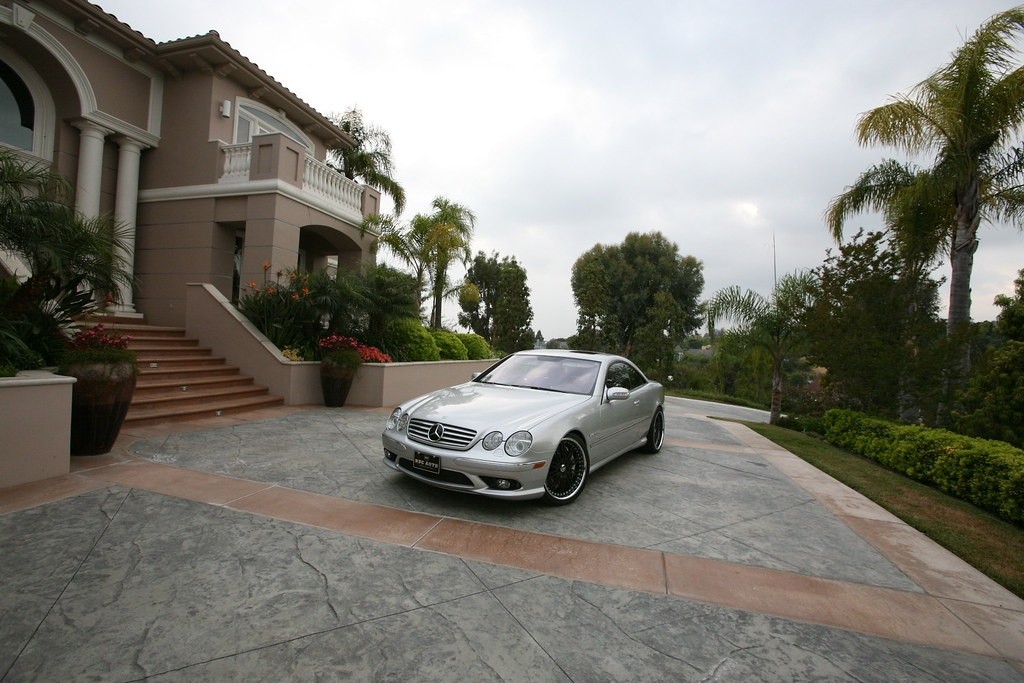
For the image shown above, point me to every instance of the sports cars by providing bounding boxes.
[379,349,666,506]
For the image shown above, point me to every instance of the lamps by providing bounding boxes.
[322,159,326,165]
[219,99,231,119]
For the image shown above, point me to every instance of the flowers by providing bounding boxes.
[69,321,136,364]
[318,333,358,354]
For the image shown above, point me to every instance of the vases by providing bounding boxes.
[57,360,136,457]
[319,348,361,407]
